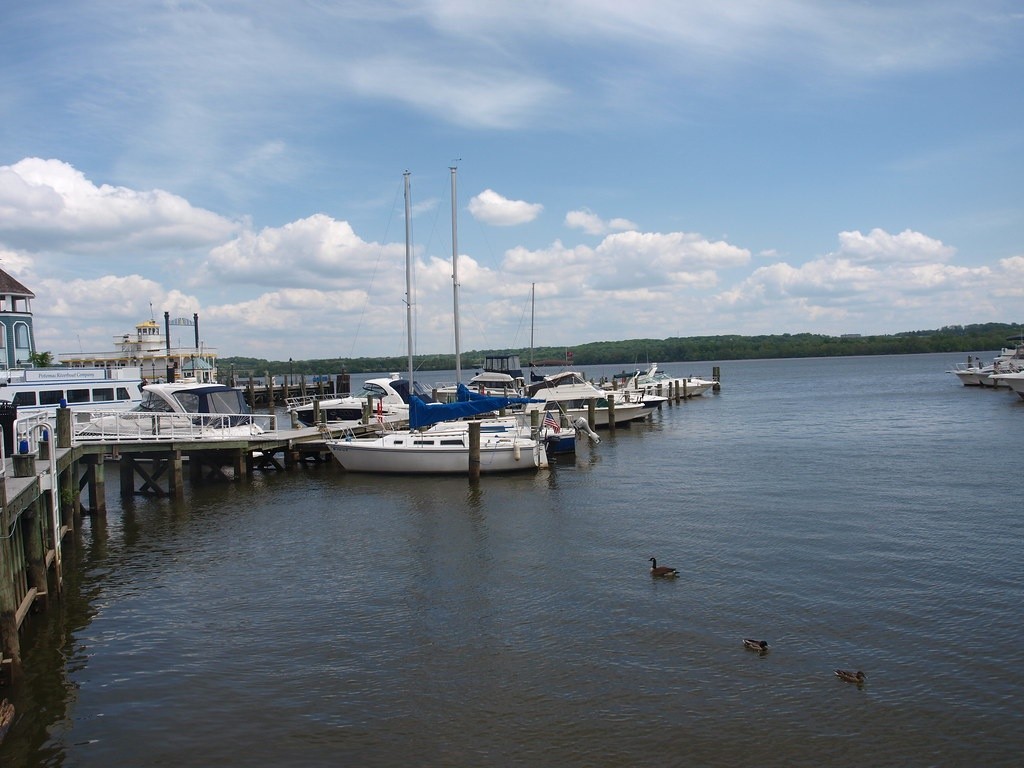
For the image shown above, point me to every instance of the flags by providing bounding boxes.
[544,411,560,433]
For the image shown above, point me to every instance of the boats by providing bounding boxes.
[283,164,719,476]
[946,332,1024,403]
[78,383,267,462]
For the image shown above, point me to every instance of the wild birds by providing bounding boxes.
[742,638,771,652]
[648,556,680,579]
[835,668,867,686]
